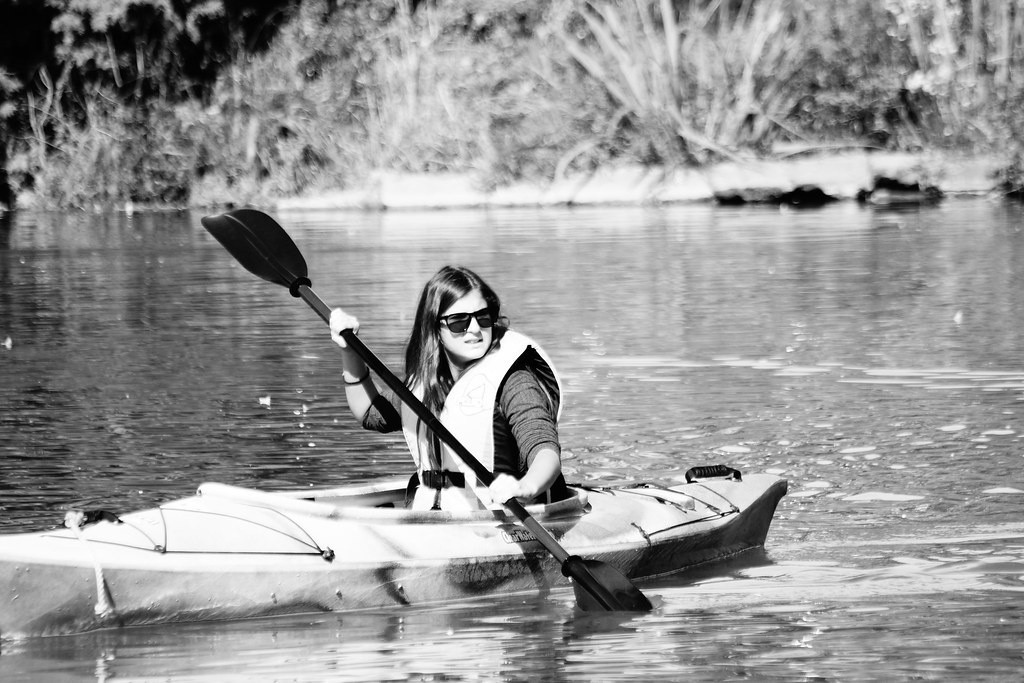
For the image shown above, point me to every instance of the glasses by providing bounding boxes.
[439,307,495,333]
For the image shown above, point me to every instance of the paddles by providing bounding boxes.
[201,207,655,612]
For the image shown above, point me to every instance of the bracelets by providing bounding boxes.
[342,367,370,385]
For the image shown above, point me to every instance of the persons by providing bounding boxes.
[329,265,566,512]
